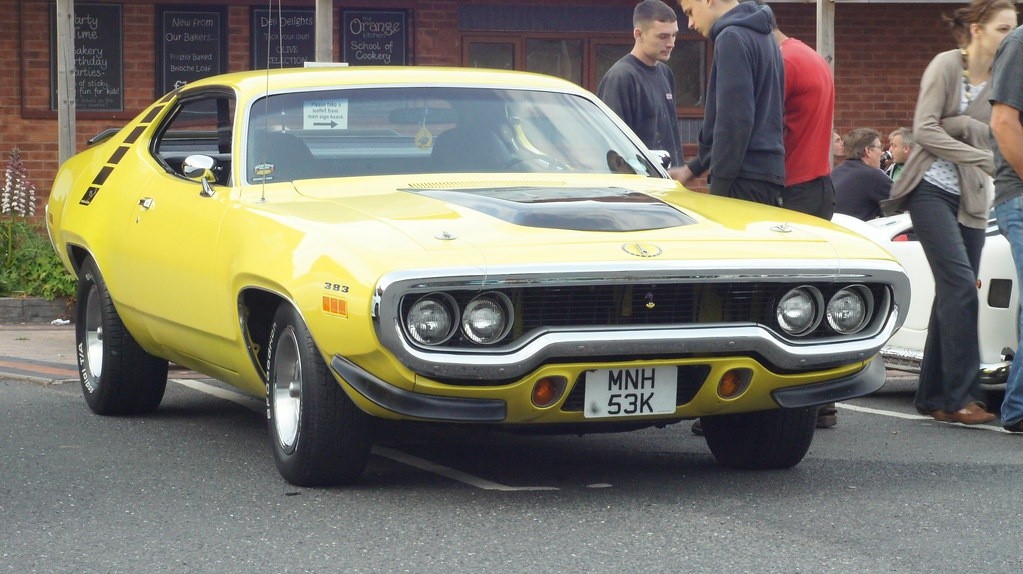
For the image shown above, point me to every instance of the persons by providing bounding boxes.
[596,0,685,180]
[987,25,1023,433]
[830,126,917,222]
[882,1,1018,433]
[667,0,833,221]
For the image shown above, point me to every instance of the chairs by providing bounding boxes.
[427,126,536,174]
[225,132,318,187]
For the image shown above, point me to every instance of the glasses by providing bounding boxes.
[869,145,884,151]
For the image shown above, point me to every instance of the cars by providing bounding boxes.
[45,62,913,487]
[825,210,1021,388]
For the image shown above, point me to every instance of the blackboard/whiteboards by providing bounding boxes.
[342,10,408,65]
[49,2,124,112]
[249,3,316,71]
[154,3,228,101]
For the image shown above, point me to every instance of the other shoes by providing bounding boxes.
[1003,419,1023,432]
[945,401,995,424]
[816,403,837,427]
[692,417,704,435]
[929,409,947,421]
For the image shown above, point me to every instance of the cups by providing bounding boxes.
[880,150,892,165]
